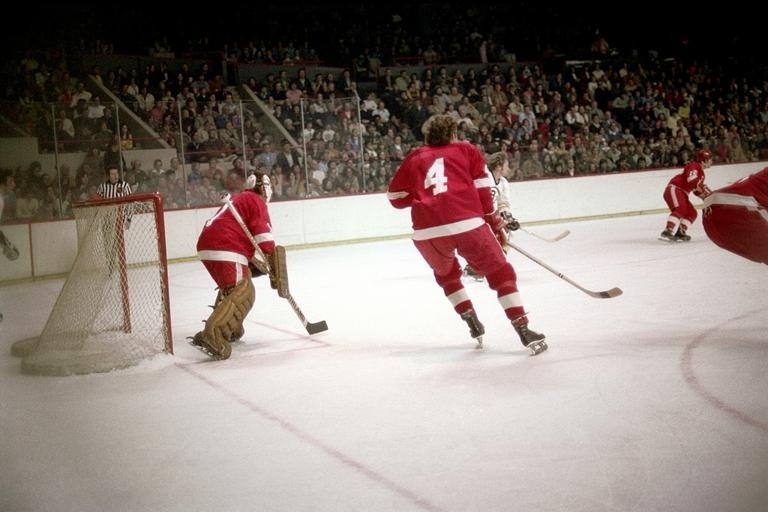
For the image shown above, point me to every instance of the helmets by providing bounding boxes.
[245,172,273,204]
[696,149,713,162]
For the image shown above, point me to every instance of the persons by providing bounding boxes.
[655,149,715,245]
[94,166,134,281]
[385,111,546,350]
[699,165,767,268]
[1,19,767,228]
[185,168,291,360]
[460,149,520,283]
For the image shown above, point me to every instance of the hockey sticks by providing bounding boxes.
[506,242,624,298]
[220,192,328,334]
[518,226,569,245]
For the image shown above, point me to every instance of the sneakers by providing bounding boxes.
[661,230,692,241]
[194,332,231,354]
[471,324,484,338]
[518,327,545,348]
[464,265,479,276]
